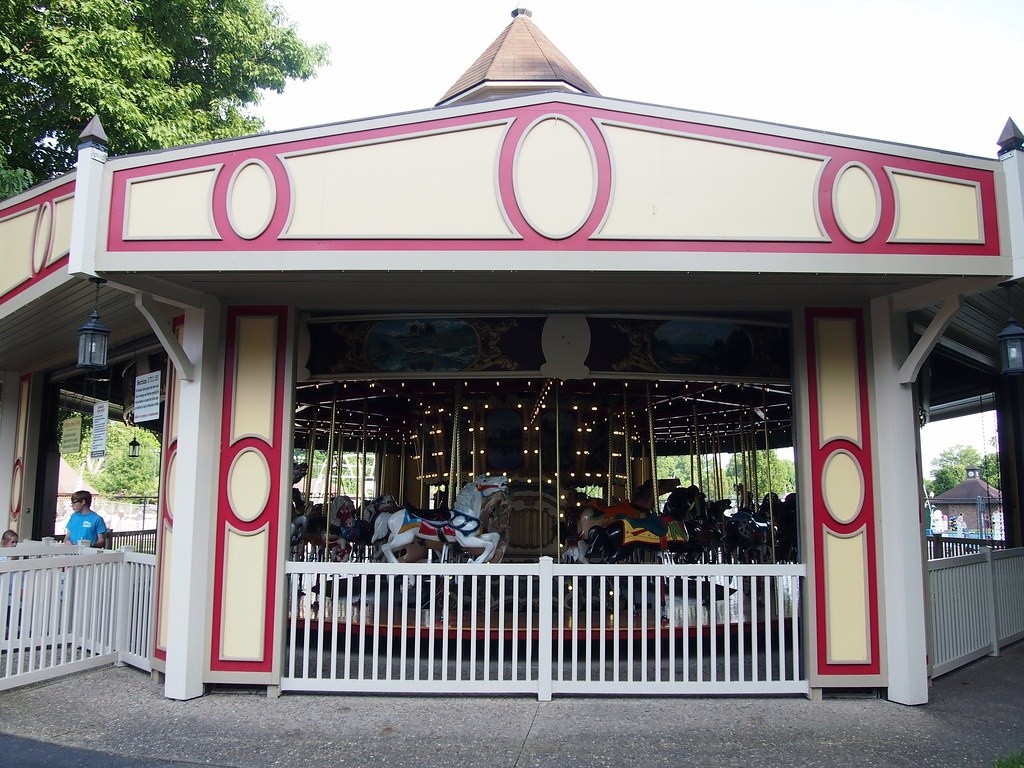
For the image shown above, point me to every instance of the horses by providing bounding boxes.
[553,479,796,624]
[290,462,511,595]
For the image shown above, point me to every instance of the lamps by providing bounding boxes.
[128,438,140,457]
[996,281,1024,376]
[75,278,111,371]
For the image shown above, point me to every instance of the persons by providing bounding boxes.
[63,491,106,567]
[0,531,24,640]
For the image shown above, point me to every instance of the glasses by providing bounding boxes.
[71,500,80,506]
